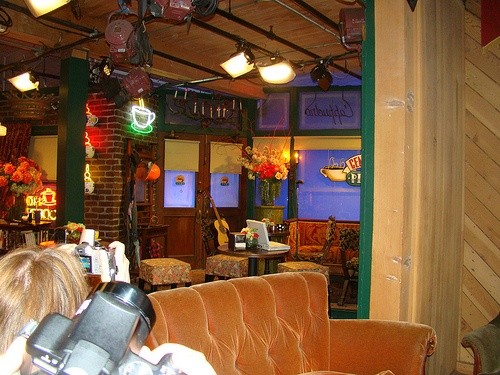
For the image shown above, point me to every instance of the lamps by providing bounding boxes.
[219,42,257,78]
[311,64,334,91]
[258,53,296,85]
[0,0,195,137]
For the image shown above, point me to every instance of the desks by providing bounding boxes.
[218,240,290,276]
[0,219,52,254]
[254,205,285,226]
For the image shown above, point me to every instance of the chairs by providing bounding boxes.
[338,227,359,304]
[461,311,500,375]
[290,215,337,264]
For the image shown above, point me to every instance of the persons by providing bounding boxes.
[0,246,89,375]
[0,315,218,375]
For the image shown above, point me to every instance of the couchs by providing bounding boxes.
[284,218,359,277]
[143,271,438,375]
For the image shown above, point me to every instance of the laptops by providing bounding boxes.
[246,219,290,251]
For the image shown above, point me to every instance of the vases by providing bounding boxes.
[260,181,276,206]
[246,240,258,250]
[14,194,25,220]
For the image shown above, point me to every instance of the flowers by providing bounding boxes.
[238,144,290,186]
[0,156,45,195]
[241,228,258,239]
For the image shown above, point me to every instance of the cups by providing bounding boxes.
[270,225,276,233]
[34,211,41,224]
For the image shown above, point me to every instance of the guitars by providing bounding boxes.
[207,194,231,247]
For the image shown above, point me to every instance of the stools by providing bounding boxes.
[205,254,274,280]
[279,261,331,319]
[138,257,193,294]
